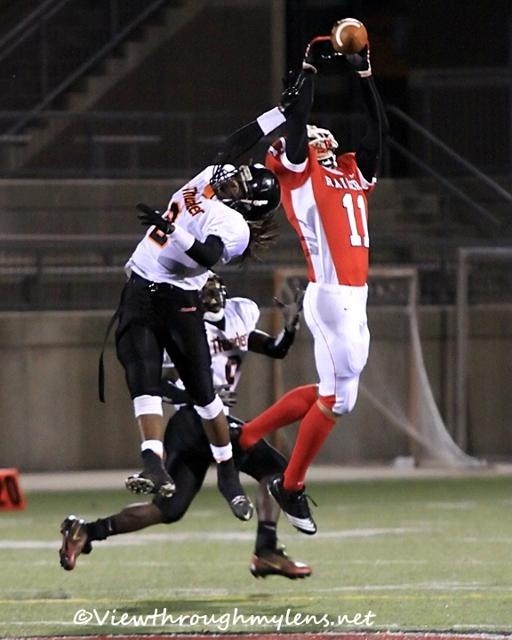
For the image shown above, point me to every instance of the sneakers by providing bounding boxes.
[58,514,92,571]
[266,472,318,535]
[228,422,248,473]
[251,554,311,579]
[216,478,253,521]
[126,469,176,498]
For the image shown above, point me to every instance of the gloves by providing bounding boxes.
[304,35,332,67]
[345,39,372,78]
[278,70,307,114]
[136,201,172,235]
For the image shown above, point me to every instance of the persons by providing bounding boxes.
[57,270,312,580]
[227,37,387,536]
[112,67,306,521]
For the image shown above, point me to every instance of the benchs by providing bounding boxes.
[1,172,511,308]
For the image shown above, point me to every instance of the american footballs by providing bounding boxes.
[332,18,368,54]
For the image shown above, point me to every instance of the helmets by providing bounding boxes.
[196,268,229,311]
[210,158,281,221]
[306,123,339,168]
